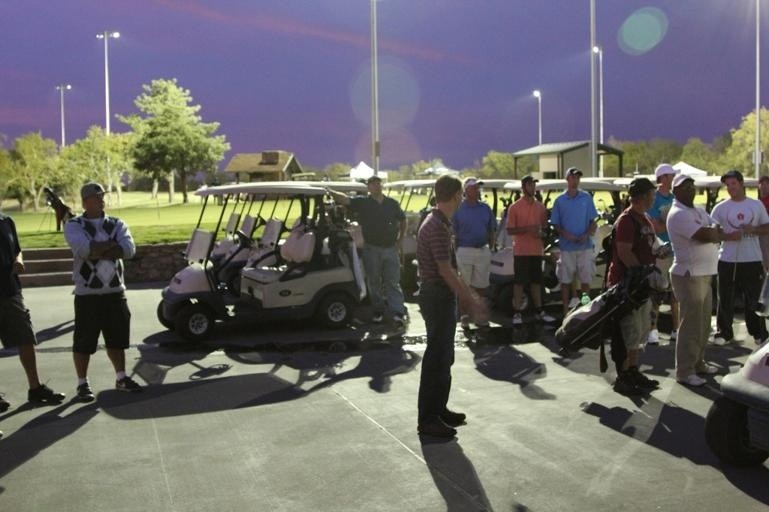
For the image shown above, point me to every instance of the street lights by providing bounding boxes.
[95,30,120,196]
[529,88,545,147]
[591,45,606,181]
[55,82,71,147]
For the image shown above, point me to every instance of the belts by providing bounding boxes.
[371,242,395,248]
[468,242,489,248]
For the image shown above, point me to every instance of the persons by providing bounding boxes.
[646,164,682,344]
[608,178,660,394]
[507,176,556,325]
[452,177,497,330]
[756,176,769,212]
[64,183,142,400]
[710,170,769,344]
[552,167,596,318]
[666,176,726,386]
[416,176,485,436]
[1,213,66,412]
[326,177,407,327]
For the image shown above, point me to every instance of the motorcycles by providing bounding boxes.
[158,182,368,342]
[704,338,769,466]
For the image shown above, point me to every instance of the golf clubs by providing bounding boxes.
[631,264,662,305]
[727,224,743,330]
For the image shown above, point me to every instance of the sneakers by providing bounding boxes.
[439,406,466,422]
[416,417,457,439]
[615,374,647,395]
[677,372,707,386]
[373,312,385,323]
[26,385,66,402]
[634,374,660,388]
[0,395,11,409]
[1,347,18,356]
[535,312,557,322]
[77,382,95,400]
[648,328,660,345]
[714,337,731,346]
[513,312,522,325]
[115,377,142,392]
[462,315,470,329]
[393,312,404,324]
[475,319,490,326]
[671,329,678,340]
[697,360,718,373]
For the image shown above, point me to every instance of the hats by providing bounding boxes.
[670,174,695,191]
[628,178,663,194]
[720,170,743,183]
[521,176,539,184]
[759,176,769,183]
[567,167,584,177]
[81,183,111,199]
[464,177,485,189]
[368,176,382,184]
[656,164,682,177]
